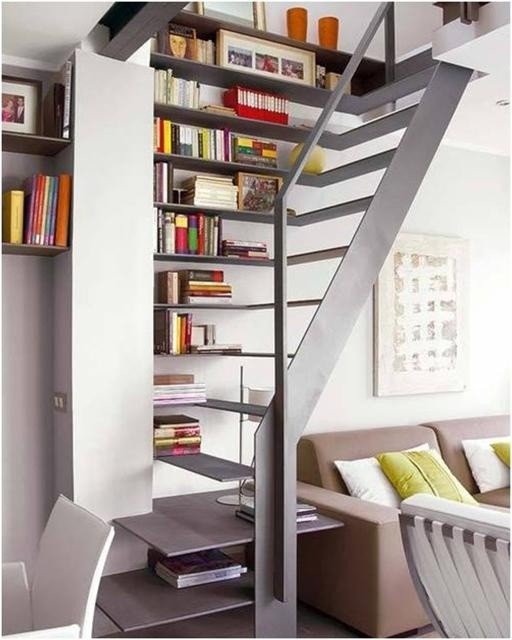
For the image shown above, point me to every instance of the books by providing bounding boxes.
[153,374,207,405]
[154,270,233,305]
[41,58,75,141]
[2,167,73,248]
[152,160,240,209]
[154,551,249,592]
[153,206,268,261]
[234,501,317,529]
[153,415,201,456]
[152,67,291,125]
[154,117,278,168]
[154,310,242,354]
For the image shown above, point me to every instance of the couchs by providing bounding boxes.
[297,414,511,638]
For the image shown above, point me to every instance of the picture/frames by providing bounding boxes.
[2,59,74,139]
[373,229,468,398]
[215,28,317,87]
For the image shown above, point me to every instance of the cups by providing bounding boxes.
[287,7,338,50]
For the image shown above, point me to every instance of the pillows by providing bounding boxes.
[334,436,511,513]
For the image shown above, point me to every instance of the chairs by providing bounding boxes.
[2,493,115,640]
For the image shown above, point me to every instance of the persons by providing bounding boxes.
[3,98,16,122]
[15,95,24,122]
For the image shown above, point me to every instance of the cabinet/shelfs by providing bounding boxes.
[155,6,387,452]
[87,489,347,634]
